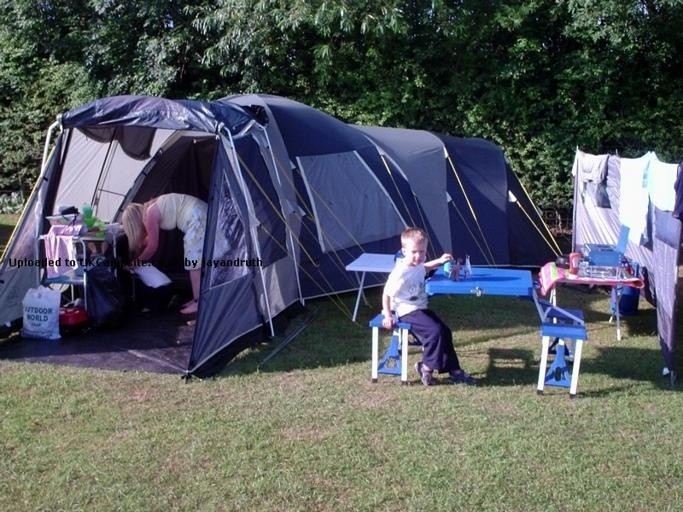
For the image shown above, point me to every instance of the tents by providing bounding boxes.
[0,91,565,384]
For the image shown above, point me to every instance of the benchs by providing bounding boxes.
[370,293,413,386]
[537,300,586,396]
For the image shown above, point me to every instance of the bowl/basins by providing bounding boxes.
[82,201,106,239]
[555,253,569,268]
[582,265,614,277]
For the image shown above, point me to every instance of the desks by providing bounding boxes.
[425,263,545,324]
[538,259,643,343]
[344,252,427,321]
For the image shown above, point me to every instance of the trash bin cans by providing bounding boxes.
[556,255,569,287]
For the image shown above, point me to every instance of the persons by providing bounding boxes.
[383,229,482,386]
[121,193,209,315]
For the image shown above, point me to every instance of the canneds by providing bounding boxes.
[443,259,455,277]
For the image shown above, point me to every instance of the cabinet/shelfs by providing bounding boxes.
[36,223,140,330]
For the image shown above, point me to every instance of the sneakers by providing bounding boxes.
[415,362,432,386]
[448,371,478,384]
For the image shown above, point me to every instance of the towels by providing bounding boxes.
[44,224,87,277]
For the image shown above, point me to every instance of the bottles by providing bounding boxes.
[616,256,638,277]
[441,255,472,279]
